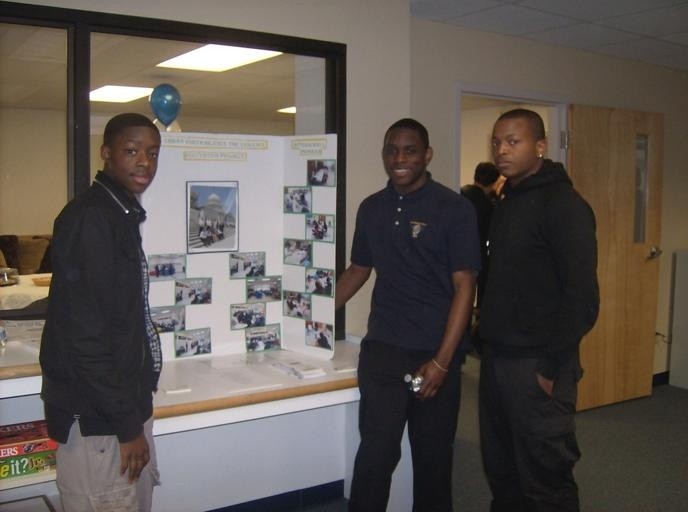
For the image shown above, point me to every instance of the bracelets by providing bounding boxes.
[430,358,452,375]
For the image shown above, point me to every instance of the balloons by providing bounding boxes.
[149,84,181,128]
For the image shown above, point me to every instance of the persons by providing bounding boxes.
[146,164,337,358]
[331,114,479,512]
[461,158,502,359]
[38,108,163,512]
[472,106,600,511]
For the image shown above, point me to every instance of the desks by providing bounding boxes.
[152,338,414,512]
[0,320,48,427]
[0,272,53,317]
